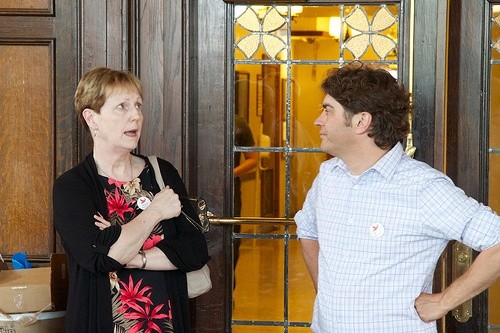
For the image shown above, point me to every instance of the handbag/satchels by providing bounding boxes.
[147,154,212,299]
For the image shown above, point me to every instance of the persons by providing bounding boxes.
[232,118,258,310]
[293,67,500,333]
[52,67,208,333]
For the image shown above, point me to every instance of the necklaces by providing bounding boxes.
[97,158,133,200]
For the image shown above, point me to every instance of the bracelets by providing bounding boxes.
[138,250,147,270]
